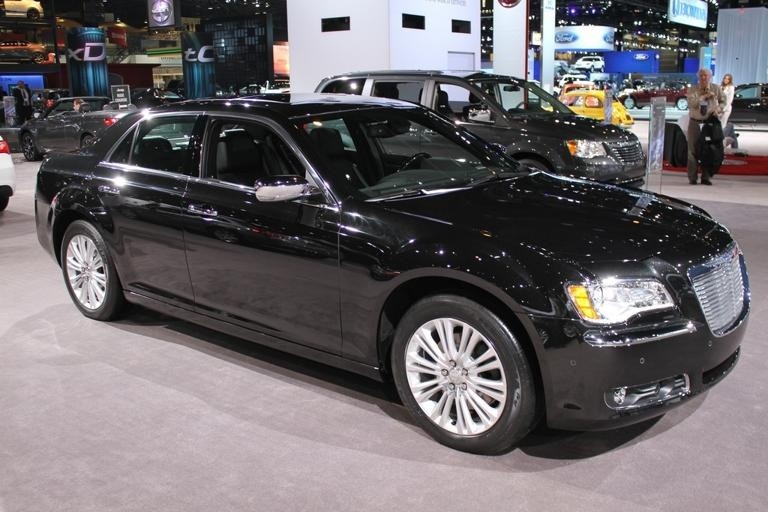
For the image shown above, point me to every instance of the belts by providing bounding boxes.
[690,117,711,123]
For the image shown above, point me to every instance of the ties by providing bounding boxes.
[700,89,707,116]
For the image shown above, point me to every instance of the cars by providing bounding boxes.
[16,96,138,162]
[572,56,607,73]
[0,0,44,21]
[0,38,49,66]
[0,133,16,212]
[31,89,758,461]
[728,81,768,110]
[311,56,693,192]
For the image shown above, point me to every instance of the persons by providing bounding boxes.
[73,99,85,112]
[719,73,734,128]
[686,68,727,186]
[12,81,33,126]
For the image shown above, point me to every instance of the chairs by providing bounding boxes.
[376,85,484,123]
[133,127,380,206]
[79,102,136,111]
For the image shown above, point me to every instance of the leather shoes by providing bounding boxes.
[689,179,712,185]
